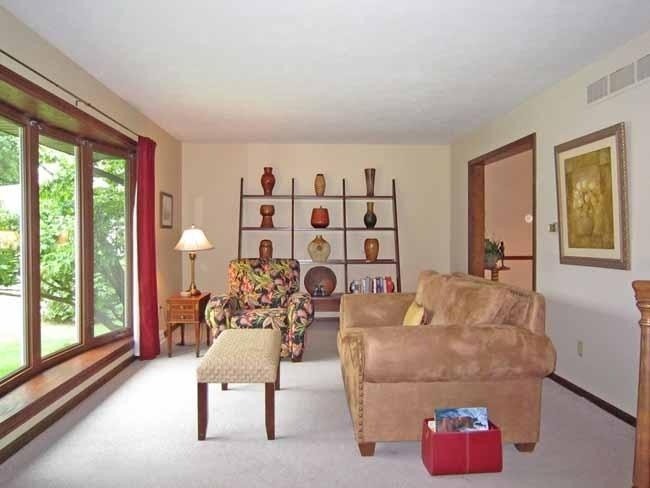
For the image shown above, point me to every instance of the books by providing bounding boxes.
[433,407,489,433]
[353,275,392,293]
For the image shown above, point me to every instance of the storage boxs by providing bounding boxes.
[421,418,503,475]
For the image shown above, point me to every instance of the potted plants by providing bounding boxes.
[486,238,502,268]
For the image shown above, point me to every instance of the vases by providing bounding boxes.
[253,167,379,262]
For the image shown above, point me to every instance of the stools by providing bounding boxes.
[196,328,282,441]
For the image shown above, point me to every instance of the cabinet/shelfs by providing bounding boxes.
[237,178,400,313]
[167,291,211,358]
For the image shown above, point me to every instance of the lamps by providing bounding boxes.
[173,223,214,296]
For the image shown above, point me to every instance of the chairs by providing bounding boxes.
[337,272,556,457]
[205,257,313,362]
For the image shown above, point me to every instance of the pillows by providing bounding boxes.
[414,270,518,326]
[403,302,428,326]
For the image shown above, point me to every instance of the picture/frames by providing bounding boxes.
[159,192,174,227]
[555,122,631,269]
